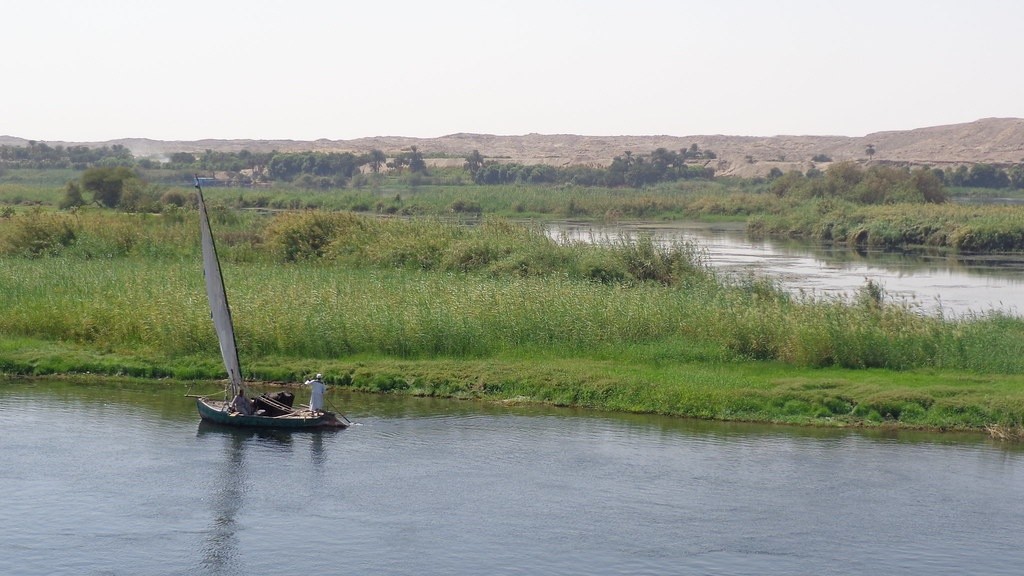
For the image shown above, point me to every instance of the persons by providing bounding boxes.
[232,389,254,415]
[305,374,325,417]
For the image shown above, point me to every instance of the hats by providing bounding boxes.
[316,374,322,378]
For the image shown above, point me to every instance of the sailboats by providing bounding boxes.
[188,173,347,428]
